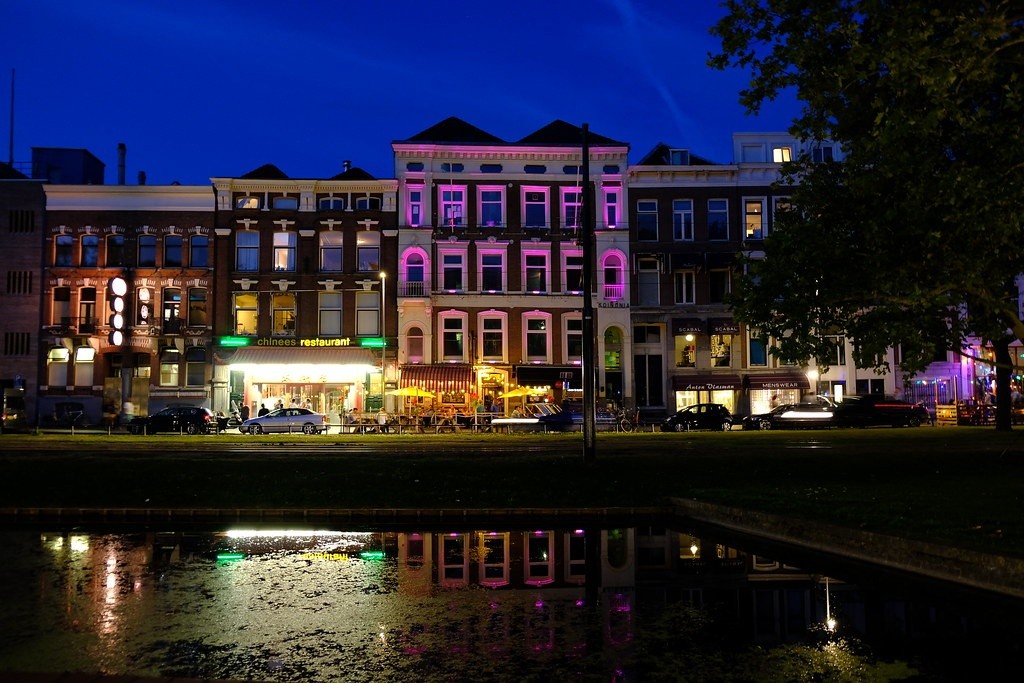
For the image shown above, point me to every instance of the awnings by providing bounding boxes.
[671,373,741,392]
[400,363,473,393]
[743,372,811,390]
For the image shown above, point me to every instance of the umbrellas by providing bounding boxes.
[498,386,548,416]
[385,386,436,418]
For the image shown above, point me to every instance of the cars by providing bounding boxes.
[660,403,733,431]
[741,403,833,429]
[238,407,327,434]
[539,403,617,432]
[127,406,211,435]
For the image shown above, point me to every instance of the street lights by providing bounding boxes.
[379,271,389,411]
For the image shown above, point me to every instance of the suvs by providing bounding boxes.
[837,395,928,427]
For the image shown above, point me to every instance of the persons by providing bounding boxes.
[415,398,499,433]
[510,405,543,434]
[768,395,778,411]
[348,407,389,434]
[802,391,817,403]
[206,397,313,432]
[916,388,1024,426]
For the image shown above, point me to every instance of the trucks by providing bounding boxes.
[16,395,103,428]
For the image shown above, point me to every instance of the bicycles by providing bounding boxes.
[618,411,638,433]
[916,395,934,427]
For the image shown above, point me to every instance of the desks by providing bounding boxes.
[353,417,379,433]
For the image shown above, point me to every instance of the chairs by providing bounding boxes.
[342,415,465,434]
